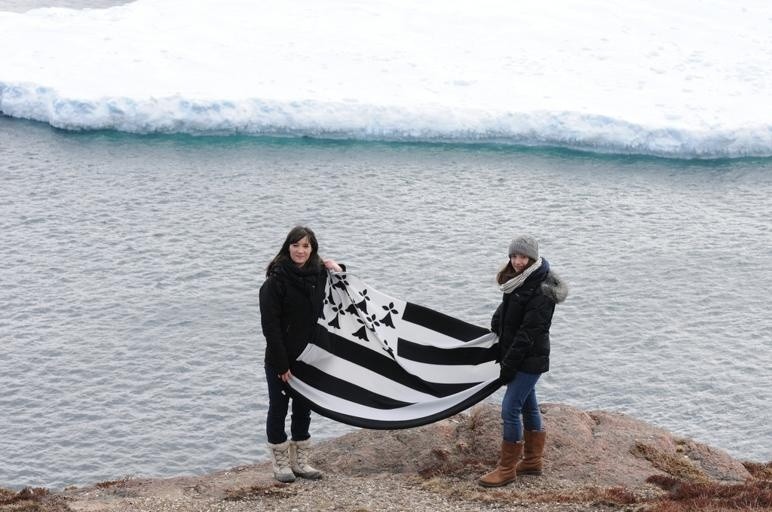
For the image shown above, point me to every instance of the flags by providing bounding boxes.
[286,260,506,433]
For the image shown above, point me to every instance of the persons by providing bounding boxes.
[259,223,349,481]
[473,235,569,486]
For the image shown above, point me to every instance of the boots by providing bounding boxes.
[267,439,296,482]
[478,439,526,487]
[515,429,547,476]
[289,436,325,479]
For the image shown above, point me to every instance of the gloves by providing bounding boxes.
[491,317,499,333]
[499,365,515,385]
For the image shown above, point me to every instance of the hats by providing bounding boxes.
[508,234,538,262]
